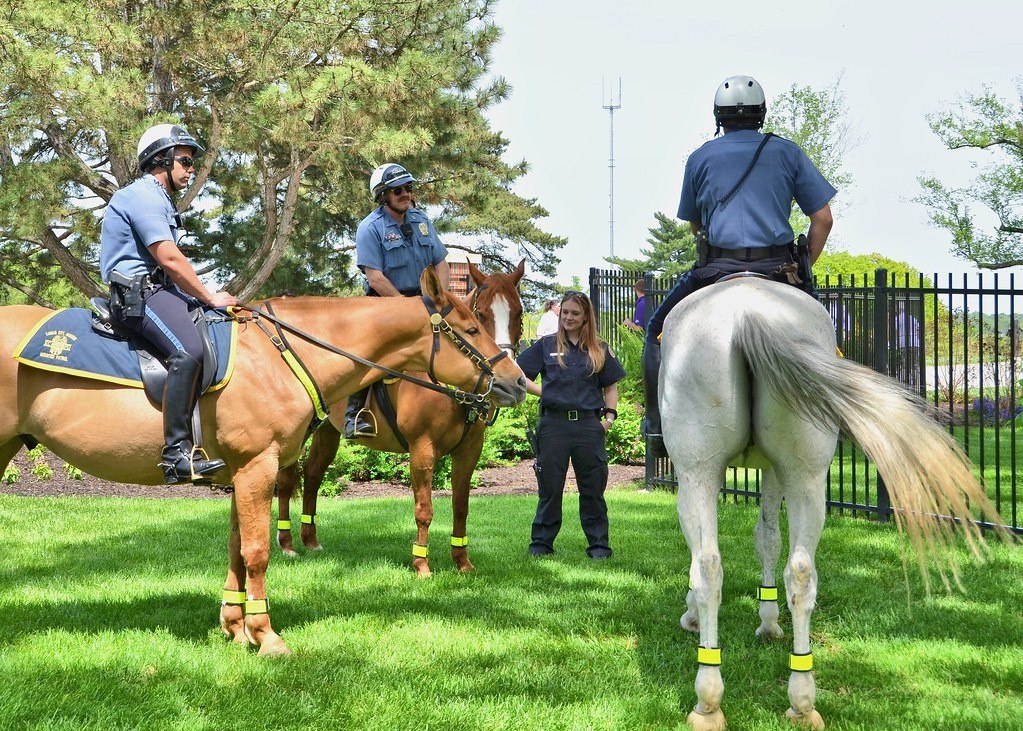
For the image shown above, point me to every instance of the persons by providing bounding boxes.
[820,291,855,342]
[624,279,659,333]
[100,124,237,485]
[516,291,627,559]
[536,299,561,340]
[888,300,920,389]
[640,76,838,458]
[342,163,450,439]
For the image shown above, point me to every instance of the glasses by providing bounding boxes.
[173,156,193,167]
[564,291,586,297]
[391,185,413,196]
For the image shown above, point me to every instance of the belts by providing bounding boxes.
[543,407,597,421]
[708,244,790,261]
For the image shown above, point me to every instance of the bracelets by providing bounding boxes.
[606,418,613,426]
[605,408,618,420]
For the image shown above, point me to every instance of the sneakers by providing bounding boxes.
[641,419,668,457]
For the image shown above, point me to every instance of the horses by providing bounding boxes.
[0,256,526,657]
[657,273,990,731]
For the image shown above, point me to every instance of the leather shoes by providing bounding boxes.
[160,443,225,485]
[344,415,373,439]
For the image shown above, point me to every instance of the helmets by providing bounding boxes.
[713,75,767,136]
[138,124,204,169]
[369,163,417,202]
